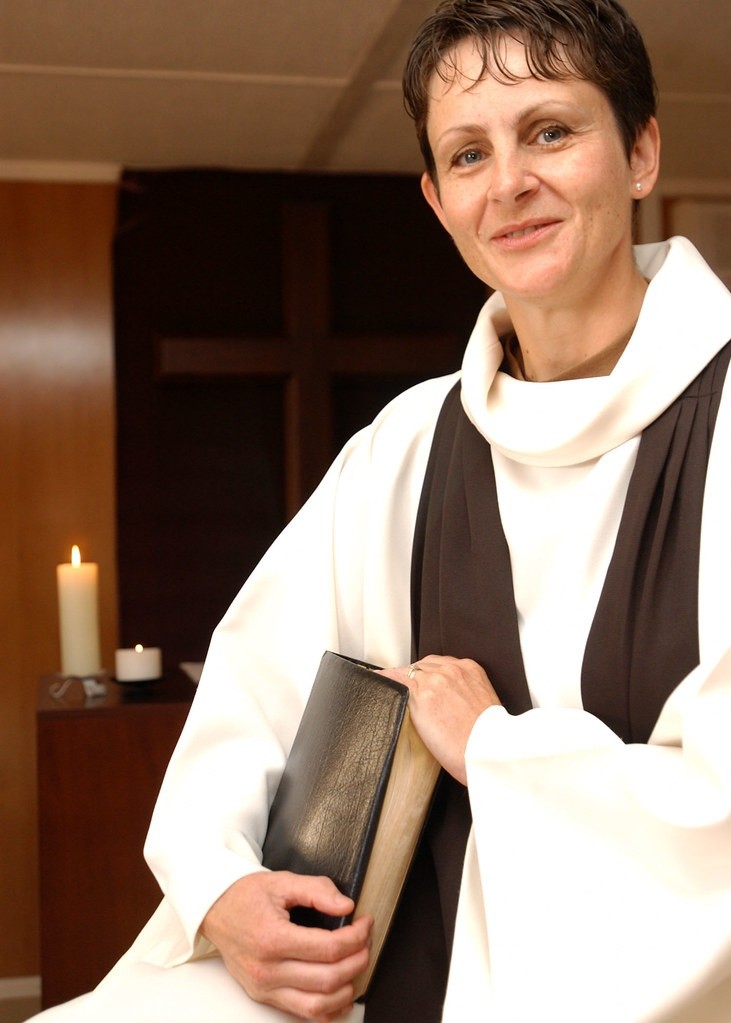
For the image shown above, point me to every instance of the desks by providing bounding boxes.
[36,673,192,1015]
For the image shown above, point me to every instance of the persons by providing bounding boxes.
[26,0,731,1023]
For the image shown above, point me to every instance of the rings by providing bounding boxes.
[408,667,420,677]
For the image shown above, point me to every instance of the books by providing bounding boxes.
[261,649,447,1005]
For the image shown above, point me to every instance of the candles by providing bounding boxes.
[115,642,163,680]
[56,545,100,677]
